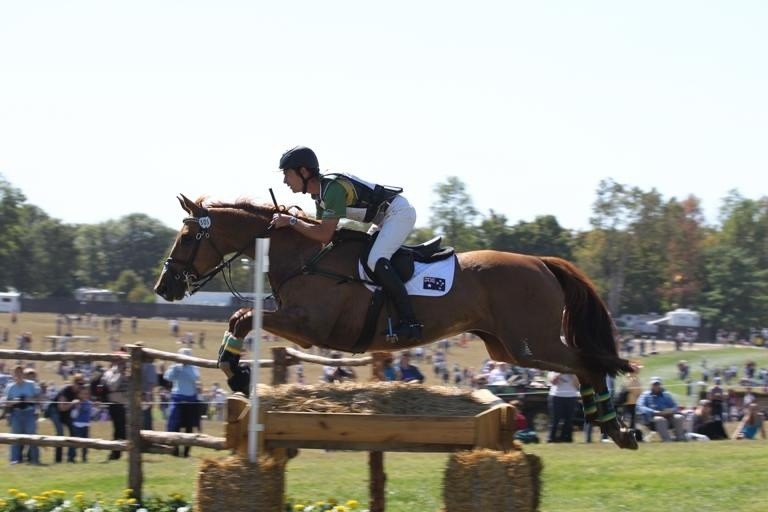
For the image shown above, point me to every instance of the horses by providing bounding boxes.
[153,192,641,451]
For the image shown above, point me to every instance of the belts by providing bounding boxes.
[378,196,396,212]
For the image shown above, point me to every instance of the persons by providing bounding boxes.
[267,338,357,384]
[0,309,252,464]
[268,144,424,347]
[621,327,768,441]
[383,333,615,442]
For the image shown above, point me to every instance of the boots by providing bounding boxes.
[373,257,422,342]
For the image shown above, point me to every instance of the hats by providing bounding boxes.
[73,373,84,385]
[651,377,661,385]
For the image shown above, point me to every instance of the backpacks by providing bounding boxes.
[91,369,107,403]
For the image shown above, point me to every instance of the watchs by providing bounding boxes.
[289,216,297,229]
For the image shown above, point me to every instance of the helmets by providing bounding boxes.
[279,145,319,169]
